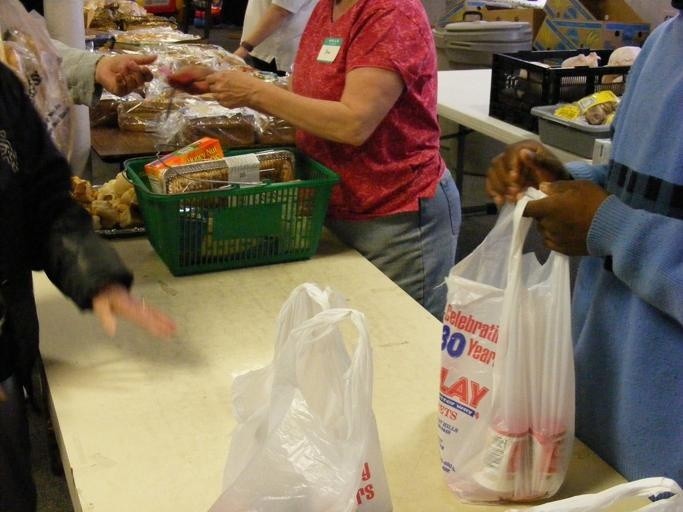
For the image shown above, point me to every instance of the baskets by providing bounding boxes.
[124,141,344,277]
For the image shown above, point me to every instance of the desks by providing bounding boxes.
[436,68,592,241]
[34,234,660,512]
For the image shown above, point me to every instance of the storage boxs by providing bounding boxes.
[531,102,612,160]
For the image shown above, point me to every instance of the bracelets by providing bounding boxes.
[241,40,254,53]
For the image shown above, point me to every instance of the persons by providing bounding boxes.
[49,0,157,109]
[233,0,316,78]
[165,0,462,323]
[487,0,682,512]
[1,60,175,512]
